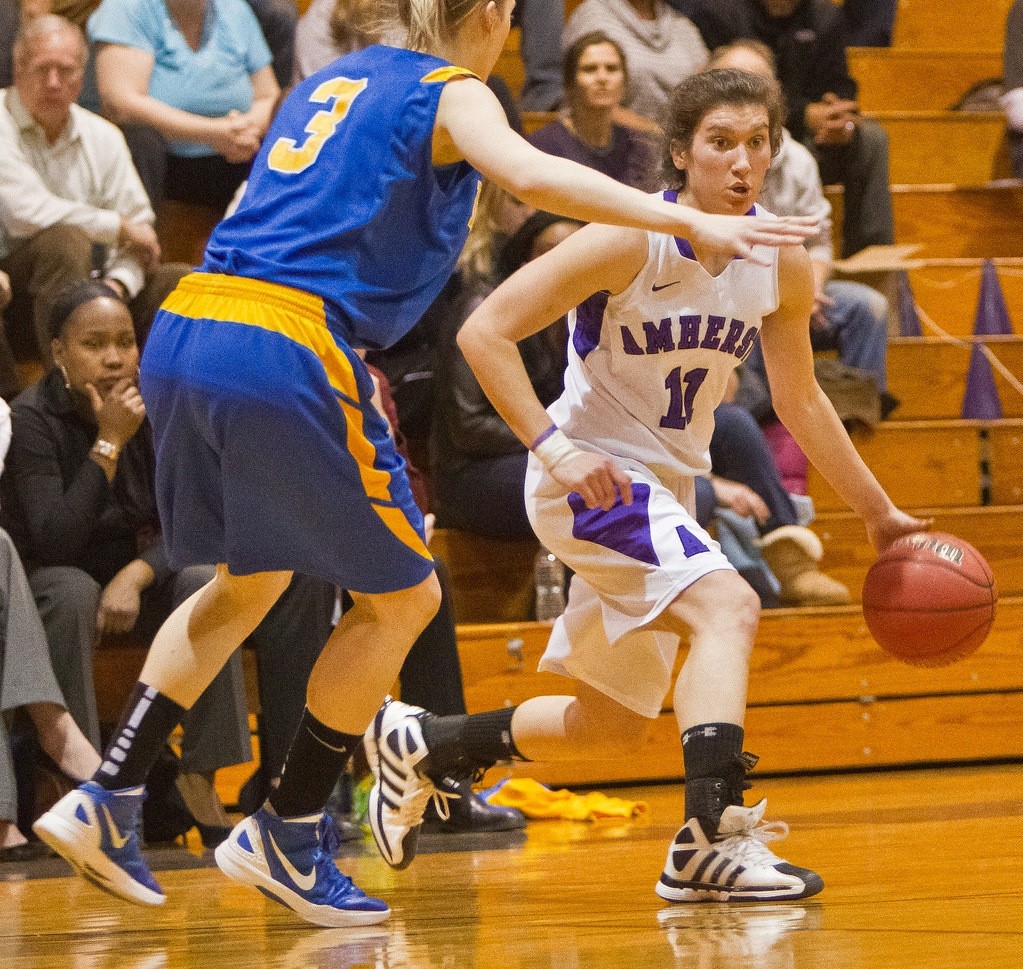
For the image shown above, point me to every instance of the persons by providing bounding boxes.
[7,283,253,852]
[0,0,893,611]
[33,0,821,930]
[364,67,938,904]
[0,400,107,857]
[256,342,527,835]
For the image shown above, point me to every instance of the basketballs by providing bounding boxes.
[861,529,1000,669]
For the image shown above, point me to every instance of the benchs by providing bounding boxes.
[0,0,1023,814]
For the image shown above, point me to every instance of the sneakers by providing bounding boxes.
[657,905,821,960]
[283,926,393,969]
[32,780,168,908]
[653,798,825,902]
[361,694,462,872]
[215,805,390,928]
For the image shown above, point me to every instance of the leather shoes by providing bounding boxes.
[422,784,526,832]
[324,806,366,841]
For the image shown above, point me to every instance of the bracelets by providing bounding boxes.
[94,438,120,462]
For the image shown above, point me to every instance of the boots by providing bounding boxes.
[756,523,851,608]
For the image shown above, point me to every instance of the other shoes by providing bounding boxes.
[0,839,33,863]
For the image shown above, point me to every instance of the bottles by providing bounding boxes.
[534,542,566,624]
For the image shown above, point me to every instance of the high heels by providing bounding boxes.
[169,779,233,850]
